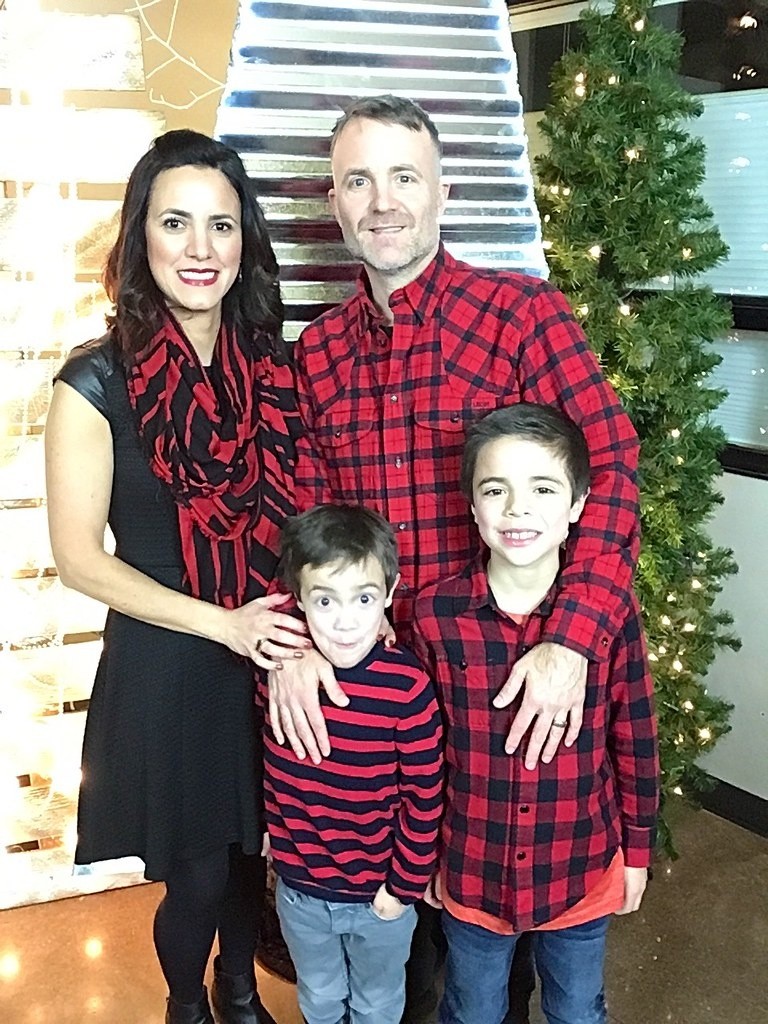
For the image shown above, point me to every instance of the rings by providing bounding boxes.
[256,638,268,650]
[552,721,567,728]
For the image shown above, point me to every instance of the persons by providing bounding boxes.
[408,402,661,1024]
[255,502,446,1024]
[44,128,296,1024]
[296,94,644,1024]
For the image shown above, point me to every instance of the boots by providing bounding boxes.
[211,957,276,1024]
[164,986,214,1024]
